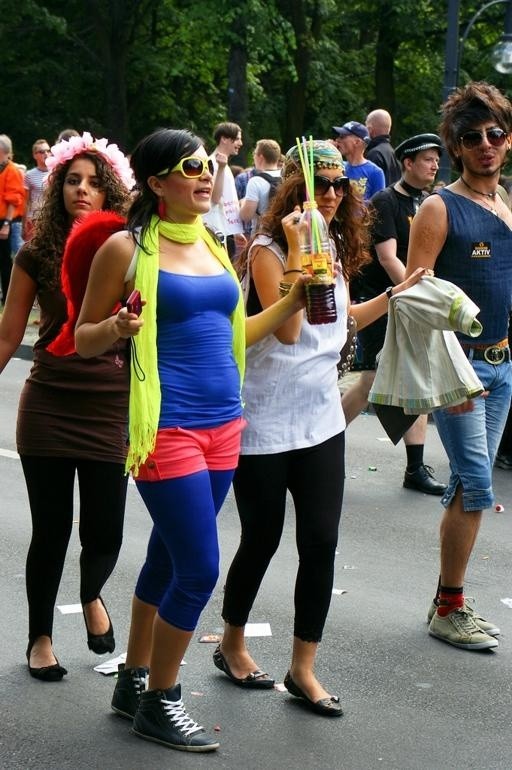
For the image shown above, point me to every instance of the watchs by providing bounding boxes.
[384,286,394,302]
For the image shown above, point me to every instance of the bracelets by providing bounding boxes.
[281,269,305,277]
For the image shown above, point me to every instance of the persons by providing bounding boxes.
[341,129,448,499]
[212,137,433,720]
[2,130,136,683]
[404,80,512,653]
[73,125,314,755]
[0,78,404,311]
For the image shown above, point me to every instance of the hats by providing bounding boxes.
[394,134,441,161]
[333,121,371,144]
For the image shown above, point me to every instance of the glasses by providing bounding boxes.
[312,175,350,198]
[158,157,214,178]
[461,129,506,149]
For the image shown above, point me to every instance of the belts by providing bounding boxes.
[461,348,511,364]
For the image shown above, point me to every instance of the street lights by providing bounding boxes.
[435,0,512,187]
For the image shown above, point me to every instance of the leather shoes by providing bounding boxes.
[214,643,274,688]
[284,670,342,716]
[403,464,448,496]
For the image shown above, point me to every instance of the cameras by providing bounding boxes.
[126,291,142,318]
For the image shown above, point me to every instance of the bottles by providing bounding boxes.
[299,201,336,324]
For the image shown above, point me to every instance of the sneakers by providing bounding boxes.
[26,646,67,681]
[429,607,498,649]
[429,599,500,636]
[131,684,220,752]
[83,595,115,654]
[111,663,150,719]
